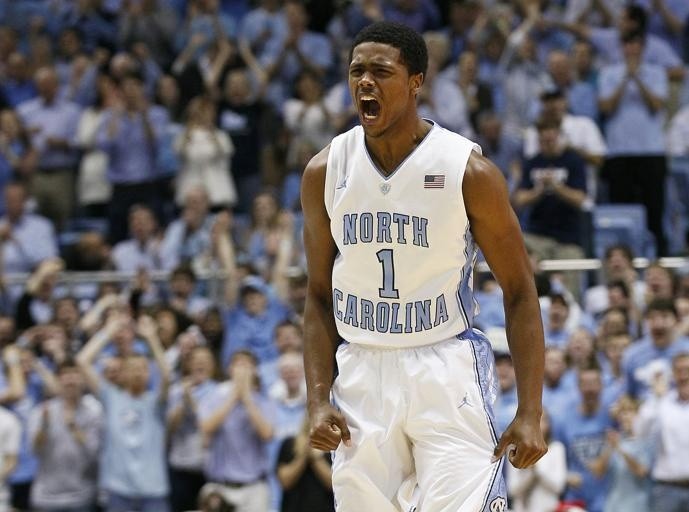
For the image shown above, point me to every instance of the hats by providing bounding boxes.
[482,326,509,355]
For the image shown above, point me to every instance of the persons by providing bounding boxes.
[1,2,360,507]
[298,21,550,509]
[385,4,687,511]
